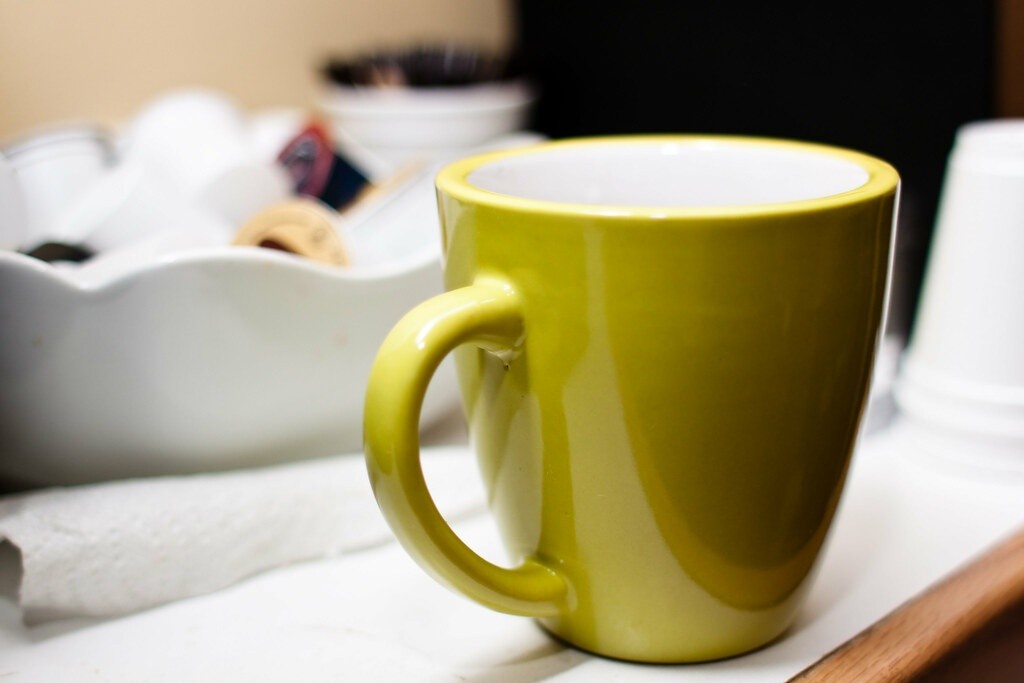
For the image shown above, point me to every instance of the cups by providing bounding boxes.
[364,135,901,668]
[313,93,528,178]
[895,121,1024,445]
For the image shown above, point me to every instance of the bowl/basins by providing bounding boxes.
[0,133,551,486]
